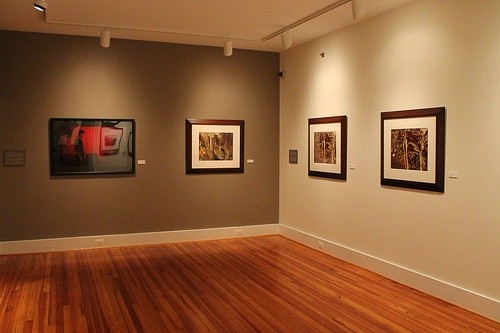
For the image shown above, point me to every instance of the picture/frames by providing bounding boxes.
[185,119,244,174]
[308,116,346,181]
[381,107,445,193]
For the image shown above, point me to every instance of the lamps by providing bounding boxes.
[224,38,232,56]
[281,30,292,50]
[352,0,365,21]
[33,0,48,12]
[100,27,110,48]
